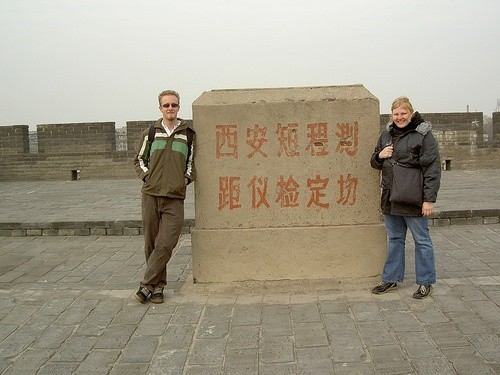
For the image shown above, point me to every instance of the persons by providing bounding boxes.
[370,96,440,299]
[134,89,195,304]
